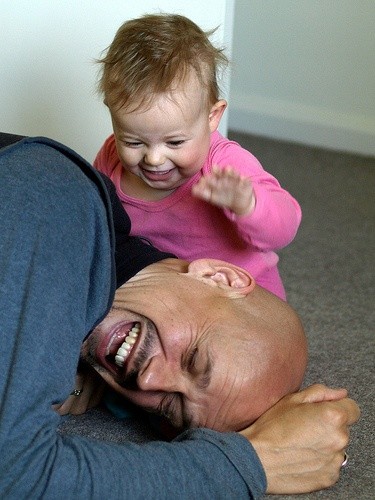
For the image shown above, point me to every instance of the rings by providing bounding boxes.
[340,453,349,468]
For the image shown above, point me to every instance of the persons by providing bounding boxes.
[1,132,361,500]
[92,14,301,301]
